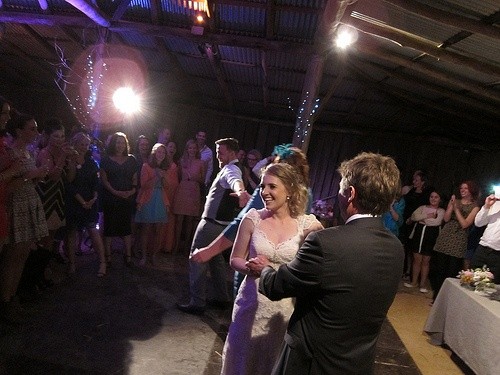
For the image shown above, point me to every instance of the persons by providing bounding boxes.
[245,152,405,375]
[1,95,500,325]
[221,162,326,375]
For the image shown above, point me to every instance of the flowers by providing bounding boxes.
[456,265,494,295]
[311,199,334,220]
[270,142,295,160]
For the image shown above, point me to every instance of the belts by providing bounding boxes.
[206,218,227,227]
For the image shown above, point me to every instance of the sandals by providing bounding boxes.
[96,262,108,277]
[68,261,78,274]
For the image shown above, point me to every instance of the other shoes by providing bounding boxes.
[176,303,205,315]
[126,255,132,266]
[404,282,419,287]
[107,254,113,265]
[207,300,232,310]
[420,286,428,292]
[136,245,156,264]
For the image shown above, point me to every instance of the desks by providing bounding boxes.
[423,278,500,375]
